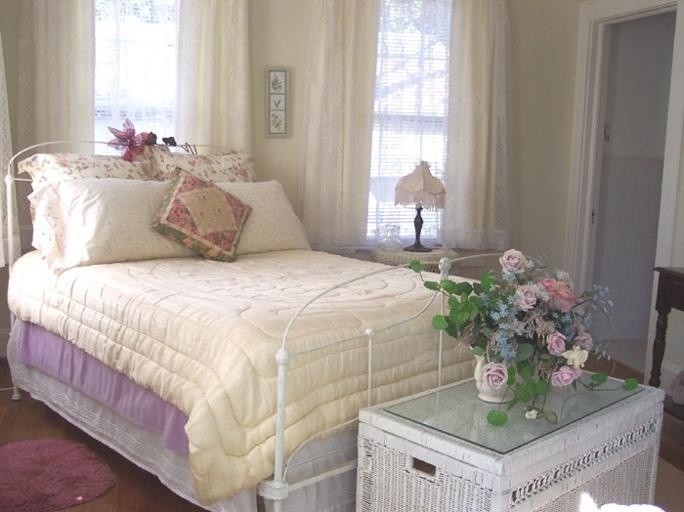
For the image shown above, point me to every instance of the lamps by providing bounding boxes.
[393,158,449,250]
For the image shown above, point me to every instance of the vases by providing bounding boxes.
[466,343,523,402]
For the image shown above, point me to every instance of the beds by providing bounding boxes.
[5,144,518,512]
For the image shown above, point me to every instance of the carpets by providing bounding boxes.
[1,438,116,511]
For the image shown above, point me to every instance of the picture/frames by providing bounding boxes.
[263,65,290,137]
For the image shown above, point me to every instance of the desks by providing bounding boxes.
[372,247,459,276]
[355,362,666,512]
[649,265,684,390]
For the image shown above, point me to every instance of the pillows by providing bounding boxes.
[152,168,253,263]
[219,179,313,254]
[15,152,154,181]
[154,144,257,183]
[28,177,200,276]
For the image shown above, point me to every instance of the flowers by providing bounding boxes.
[406,248,639,425]
[108,118,197,161]
